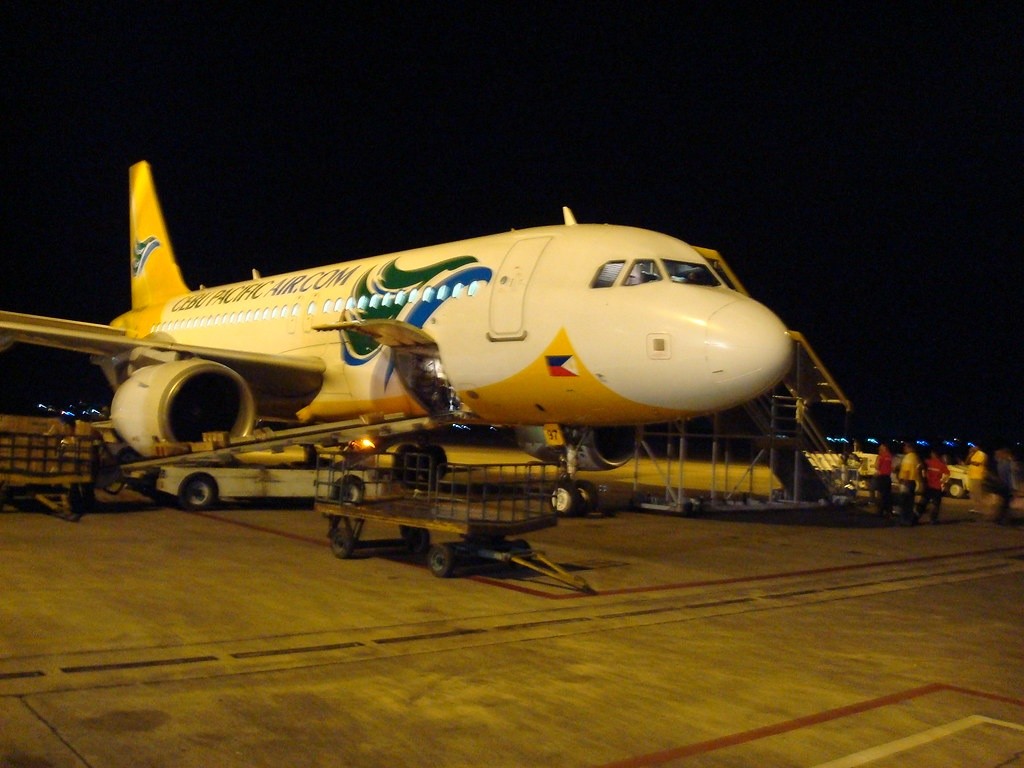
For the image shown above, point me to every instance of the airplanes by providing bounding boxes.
[2,160,795,596]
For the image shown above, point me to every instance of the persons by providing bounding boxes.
[869,443,893,518]
[994,449,1019,526]
[55,417,81,519]
[965,441,988,514]
[896,441,950,527]
